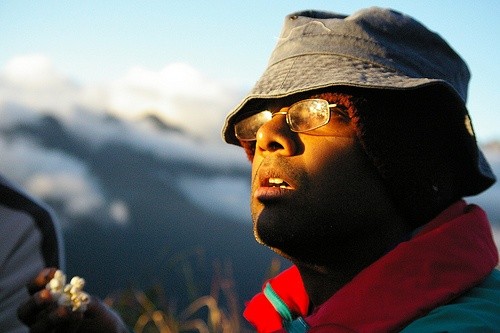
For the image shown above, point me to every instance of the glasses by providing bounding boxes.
[232,98,350,142]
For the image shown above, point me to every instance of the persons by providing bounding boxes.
[17,7,500,333]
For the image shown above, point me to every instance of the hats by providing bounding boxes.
[221,6,498,198]
